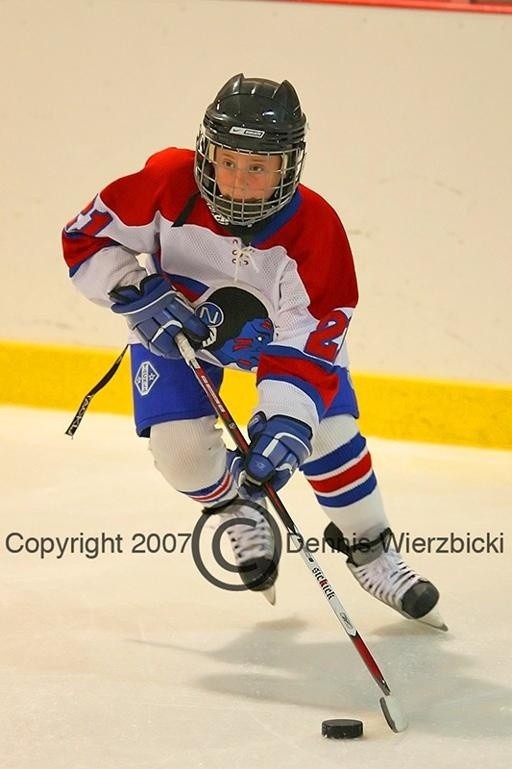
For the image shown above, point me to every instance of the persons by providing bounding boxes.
[60,72,441,621]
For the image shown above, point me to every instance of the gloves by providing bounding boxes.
[109,273,210,361]
[225,412,317,501]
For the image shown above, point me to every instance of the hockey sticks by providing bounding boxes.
[138,252,407,730]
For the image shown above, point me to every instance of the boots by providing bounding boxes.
[323,519,440,621]
[196,487,279,591]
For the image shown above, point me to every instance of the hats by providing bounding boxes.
[199,73,307,180]
